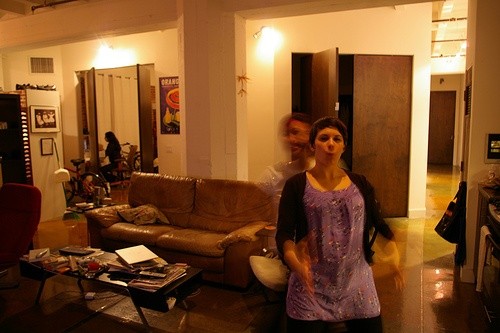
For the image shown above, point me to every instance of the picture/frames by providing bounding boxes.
[29,105,61,133]
[40,138,53,155]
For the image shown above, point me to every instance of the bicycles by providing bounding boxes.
[63,159,109,204]
[119,143,141,170]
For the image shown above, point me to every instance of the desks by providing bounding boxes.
[472,183,500,324]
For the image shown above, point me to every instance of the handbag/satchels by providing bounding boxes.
[434,189,461,243]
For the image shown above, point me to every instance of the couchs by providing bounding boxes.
[85,171,273,293]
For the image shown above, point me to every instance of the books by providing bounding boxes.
[104,244,190,293]
[59,244,95,256]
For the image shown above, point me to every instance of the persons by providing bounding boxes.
[35,112,55,127]
[249,113,404,333]
[99,132,121,182]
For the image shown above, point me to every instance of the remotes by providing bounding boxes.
[139,271,166,279]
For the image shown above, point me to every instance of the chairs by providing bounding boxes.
[0,183,41,309]
[110,145,137,189]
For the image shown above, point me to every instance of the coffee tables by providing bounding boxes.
[19,247,204,333]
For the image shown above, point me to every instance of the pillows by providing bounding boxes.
[117,203,171,225]
